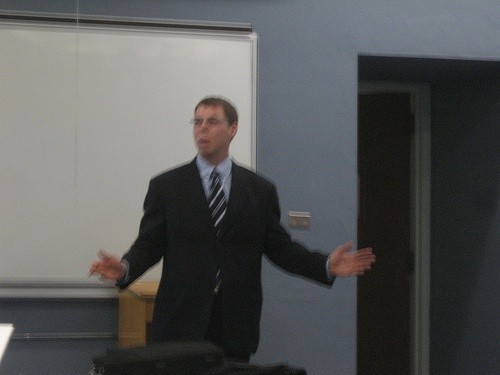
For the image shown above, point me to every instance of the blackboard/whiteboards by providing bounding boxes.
[0,8,259,304]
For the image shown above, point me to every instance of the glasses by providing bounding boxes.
[190,115,226,129]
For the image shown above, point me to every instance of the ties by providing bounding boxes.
[206,166,230,239]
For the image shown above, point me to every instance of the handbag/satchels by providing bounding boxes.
[90,340,229,375]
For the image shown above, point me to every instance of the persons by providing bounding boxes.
[88,96,376,364]
